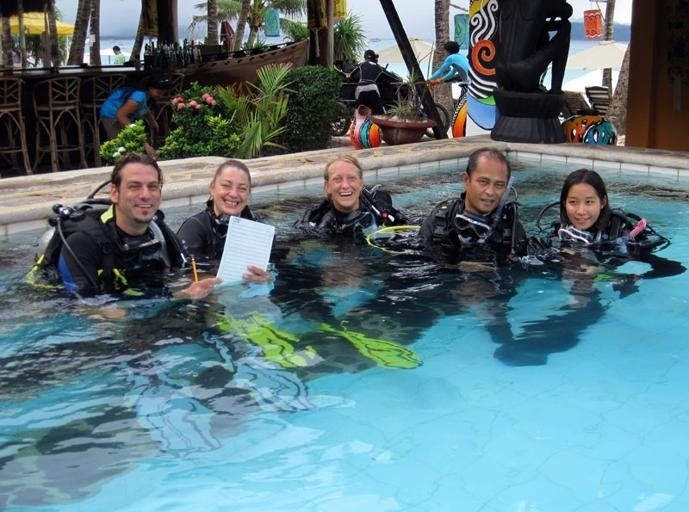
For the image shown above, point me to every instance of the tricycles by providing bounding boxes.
[326,58,450,138]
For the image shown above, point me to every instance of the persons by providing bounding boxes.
[493,1,573,97]
[529,169,652,248]
[113,45,126,66]
[418,147,532,259]
[100,68,172,162]
[428,40,470,138]
[176,160,291,262]
[49,152,271,299]
[350,49,404,116]
[293,153,411,237]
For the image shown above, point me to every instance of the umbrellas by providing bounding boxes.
[357,37,437,64]
[0,11,75,37]
[564,38,631,69]
[220,19,236,54]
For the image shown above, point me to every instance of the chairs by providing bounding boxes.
[1,72,184,175]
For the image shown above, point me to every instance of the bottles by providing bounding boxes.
[143,37,202,70]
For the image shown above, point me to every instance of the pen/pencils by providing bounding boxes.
[191,255,198,282]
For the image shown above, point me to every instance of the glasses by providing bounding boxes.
[558,222,591,247]
[453,214,491,238]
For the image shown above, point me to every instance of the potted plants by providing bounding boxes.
[372,66,439,145]
